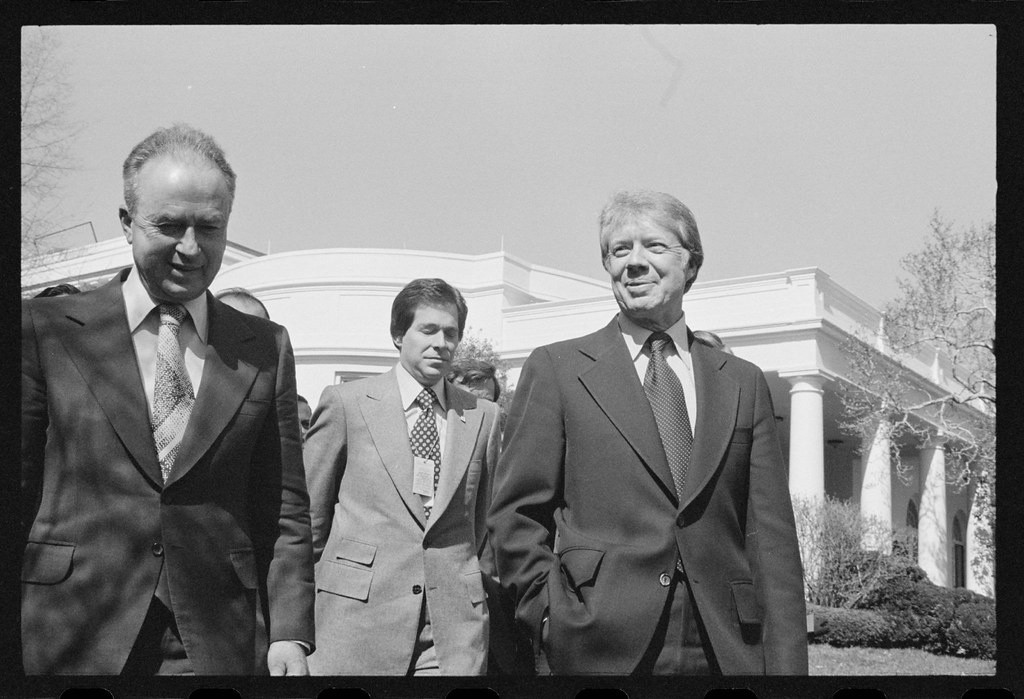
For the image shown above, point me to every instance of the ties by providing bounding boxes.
[409,388,442,521]
[643,332,693,573]
[154,303,195,614]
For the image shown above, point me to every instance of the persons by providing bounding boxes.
[32,277,500,440]
[15,123,317,675]
[298,277,499,677]
[489,190,811,676]
